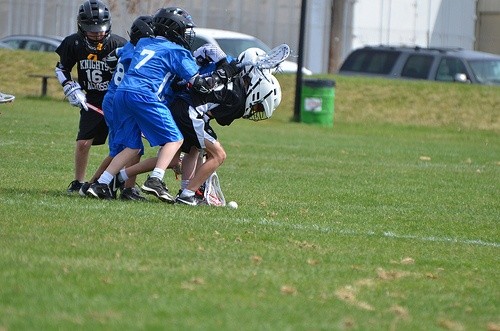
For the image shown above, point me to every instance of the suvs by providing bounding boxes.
[338,43,500,86]
[184,27,313,76]
[0,34,65,52]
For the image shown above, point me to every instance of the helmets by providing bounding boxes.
[235,47,283,119]
[130,15,154,47]
[152,7,197,50]
[77,0,112,32]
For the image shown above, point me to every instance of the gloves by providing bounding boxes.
[62,81,89,112]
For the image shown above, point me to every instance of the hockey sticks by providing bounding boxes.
[177,43,290,85]
[84,101,146,139]
[203,170,226,207]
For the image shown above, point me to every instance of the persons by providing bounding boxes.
[54,0,129,193]
[79,8,282,206]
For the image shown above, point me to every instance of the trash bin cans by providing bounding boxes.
[300,79,336,127]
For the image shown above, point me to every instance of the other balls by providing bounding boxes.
[227,201,238,210]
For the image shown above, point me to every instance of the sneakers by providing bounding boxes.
[67,175,207,206]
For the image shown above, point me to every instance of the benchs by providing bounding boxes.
[28,73,78,96]
